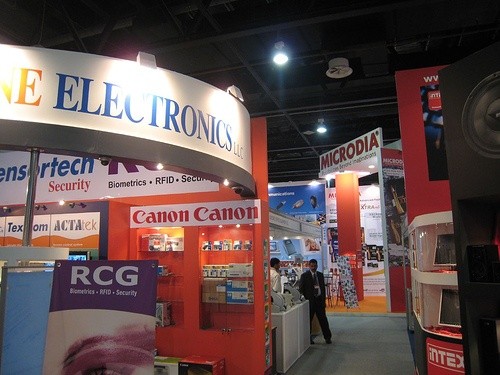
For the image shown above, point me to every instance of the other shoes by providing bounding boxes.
[310,340,314,344]
[325,339,331,344]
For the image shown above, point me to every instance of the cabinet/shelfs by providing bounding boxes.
[271,300,312,374]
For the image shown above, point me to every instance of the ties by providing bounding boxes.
[314,274,318,295]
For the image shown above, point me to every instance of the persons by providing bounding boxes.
[299,259,332,344]
[270,258,281,293]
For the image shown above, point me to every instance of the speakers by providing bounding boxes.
[438,49,500,197]
[456,245,500,375]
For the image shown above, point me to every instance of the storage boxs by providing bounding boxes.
[155,302,172,328]
[154,355,225,375]
[227,263,254,277]
[140,233,184,251]
[202,278,255,305]
[158,266,168,276]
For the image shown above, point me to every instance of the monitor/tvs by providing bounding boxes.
[438,288,462,328]
[433,234,457,266]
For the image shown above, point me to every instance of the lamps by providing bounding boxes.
[274,41,288,65]
[316,118,328,134]
